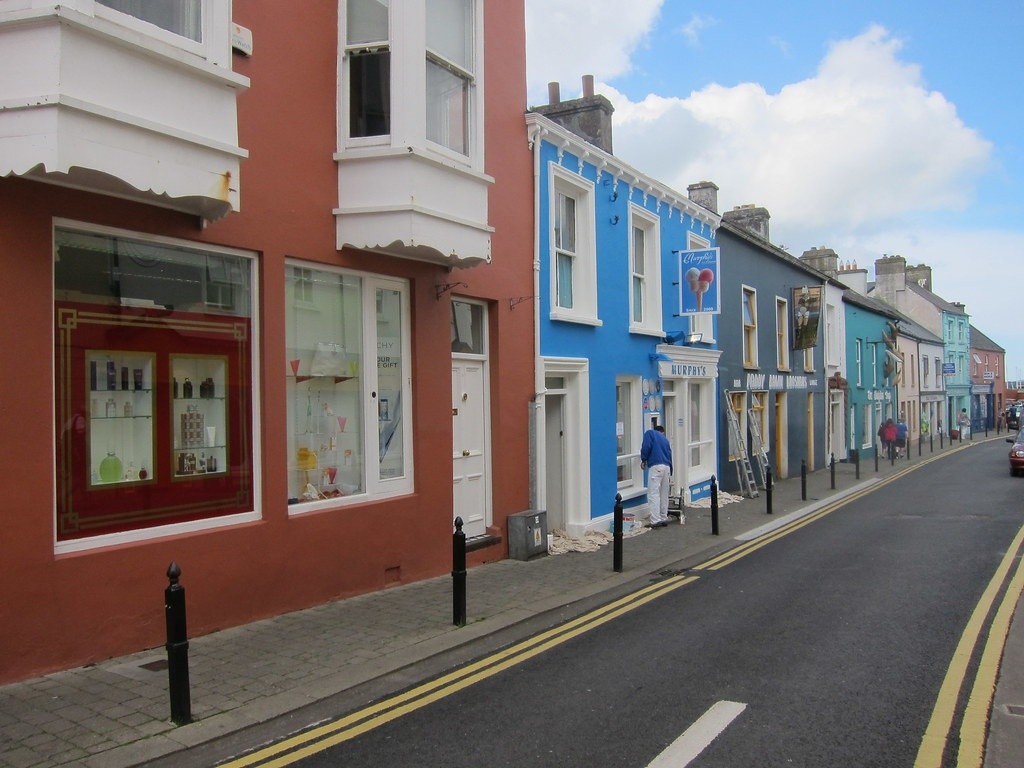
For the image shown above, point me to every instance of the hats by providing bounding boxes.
[1001,413,1005,416]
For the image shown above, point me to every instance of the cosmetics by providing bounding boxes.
[172,375,217,474]
[90,359,142,418]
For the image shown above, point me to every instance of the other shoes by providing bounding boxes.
[896,451,899,457]
[646,522,667,528]
[901,454,904,457]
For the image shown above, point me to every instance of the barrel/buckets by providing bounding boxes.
[622,513,635,533]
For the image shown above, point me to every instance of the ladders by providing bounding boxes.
[722,388,760,500]
[746,408,775,492]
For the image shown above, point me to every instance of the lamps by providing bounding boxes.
[683,333,703,346]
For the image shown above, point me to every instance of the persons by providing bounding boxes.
[997,408,1005,432]
[640,425,673,529]
[796,289,815,339]
[1010,404,1017,416]
[879,418,908,459]
[957,408,971,439]
[1020,405,1024,421]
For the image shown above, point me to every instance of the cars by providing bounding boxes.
[1006,398,1024,430]
[1006,426,1024,477]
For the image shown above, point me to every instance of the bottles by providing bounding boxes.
[139,467,147,479]
[124,402,133,417]
[173,377,178,399]
[105,398,117,418]
[183,378,192,398]
[99,451,122,483]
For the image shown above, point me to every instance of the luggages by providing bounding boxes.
[951,424,959,440]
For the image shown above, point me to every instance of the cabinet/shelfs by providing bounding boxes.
[175,397,227,452]
[90,387,153,420]
[287,371,358,497]
